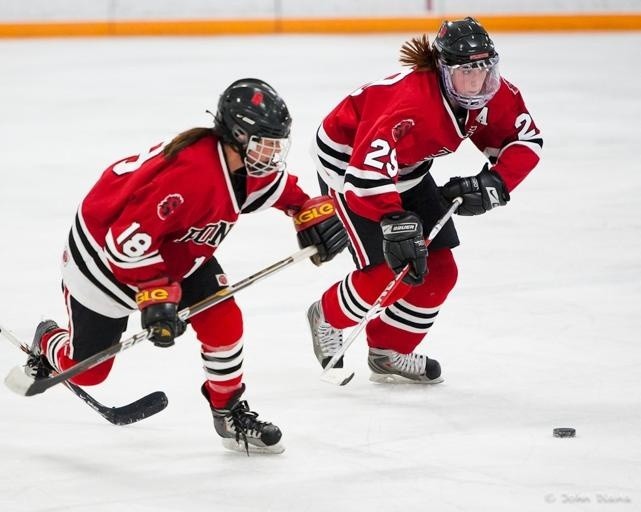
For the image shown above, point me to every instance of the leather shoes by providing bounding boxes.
[380,209,430,286]
[293,196,351,267]
[436,162,511,218]
[135,282,187,348]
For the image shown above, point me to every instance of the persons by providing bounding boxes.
[26,74,348,457]
[309,17,543,381]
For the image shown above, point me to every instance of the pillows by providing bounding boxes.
[431,15,503,112]
[205,77,293,178]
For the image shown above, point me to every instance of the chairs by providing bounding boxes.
[368,347,442,381]
[23,319,58,381]
[201,381,282,456]
[306,299,344,369]
